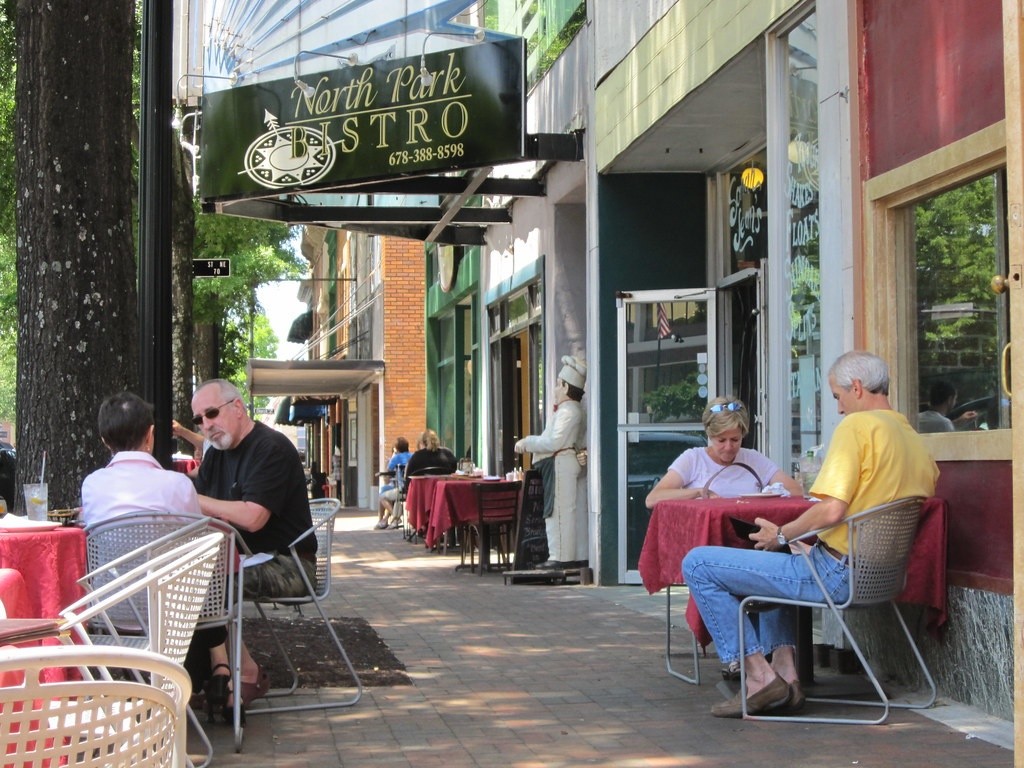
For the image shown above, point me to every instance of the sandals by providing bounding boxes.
[189,664,271,711]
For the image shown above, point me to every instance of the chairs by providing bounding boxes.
[0,494,373,768]
[739,495,947,723]
[375,465,527,577]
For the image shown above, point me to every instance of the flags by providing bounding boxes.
[655,302,670,340]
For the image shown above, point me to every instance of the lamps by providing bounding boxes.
[178,109,205,154]
[743,159,764,188]
[785,132,813,163]
[291,50,359,98]
[417,24,483,88]
[172,71,237,131]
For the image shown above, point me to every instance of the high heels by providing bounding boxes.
[220,691,247,724]
[206,664,231,702]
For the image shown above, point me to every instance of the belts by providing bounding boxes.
[284,551,317,563]
[817,538,852,565]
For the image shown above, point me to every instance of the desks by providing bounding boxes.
[174,459,202,475]
[406,473,485,544]
[0,527,84,702]
[423,480,521,571]
[637,494,951,687]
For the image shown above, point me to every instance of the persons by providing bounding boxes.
[645,395,803,507]
[403,429,458,493]
[681,350,940,717]
[514,355,587,570]
[374,437,414,529]
[188,379,318,707]
[917,381,979,433]
[172,419,212,477]
[82,392,246,723]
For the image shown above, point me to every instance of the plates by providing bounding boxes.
[739,493,783,497]
[0,518,62,532]
[483,478,501,480]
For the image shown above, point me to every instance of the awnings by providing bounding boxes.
[273,391,338,427]
[286,310,313,344]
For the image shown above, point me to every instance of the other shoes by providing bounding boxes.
[711,671,793,717]
[387,518,402,529]
[777,681,805,713]
[378,520,388,528]
[721,664,741,680]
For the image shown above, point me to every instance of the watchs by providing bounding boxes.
[776,526,788,545]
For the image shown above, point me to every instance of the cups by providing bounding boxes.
[797,456,822,499]
[23,482,49,522]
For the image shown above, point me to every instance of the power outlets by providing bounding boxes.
[0,616,70,646]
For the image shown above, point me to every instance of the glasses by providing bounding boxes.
[192,400,234,424]
[710,402,747,413]
